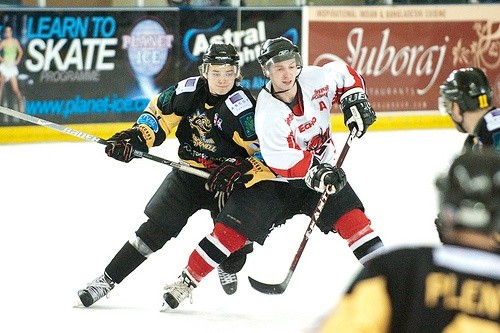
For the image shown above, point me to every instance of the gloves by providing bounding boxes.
[105,130,149,162]
[207,157,254,192]
[339,93,375,138]
[304,164,347,195]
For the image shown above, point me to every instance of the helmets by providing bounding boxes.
[202,44,240,66]
[436,152,500,233]
[257,37,300,65]
[441,68,493,111]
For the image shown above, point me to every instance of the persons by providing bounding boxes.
[441,67,500,150]
[0,25,25,112]
[316,147,500,333]
[160,38,384,312]
[74,44,279,309]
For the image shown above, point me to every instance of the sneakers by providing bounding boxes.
[160,272,199,311]
[217,265,237,295]
[74,271,115,308]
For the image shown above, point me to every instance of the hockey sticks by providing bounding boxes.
[247,126,358,295]
[0,105,211,180]
